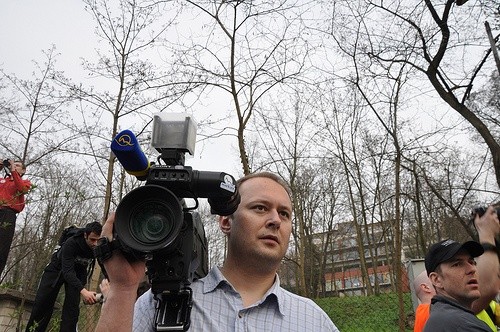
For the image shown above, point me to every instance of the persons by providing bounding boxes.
[24,222,151,332]
[0,159,30,275]
[93,173,341,332]
[414,205,500,332]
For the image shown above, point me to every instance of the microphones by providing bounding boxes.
[111,130,150,181]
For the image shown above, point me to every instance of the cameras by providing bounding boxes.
[475,206,500,224]
[3,158,11,167]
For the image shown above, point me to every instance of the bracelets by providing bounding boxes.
[482,241,499,254]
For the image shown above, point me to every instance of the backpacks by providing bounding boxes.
[50,225,84,261]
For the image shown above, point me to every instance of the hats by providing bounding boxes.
[424,239,484,275]
